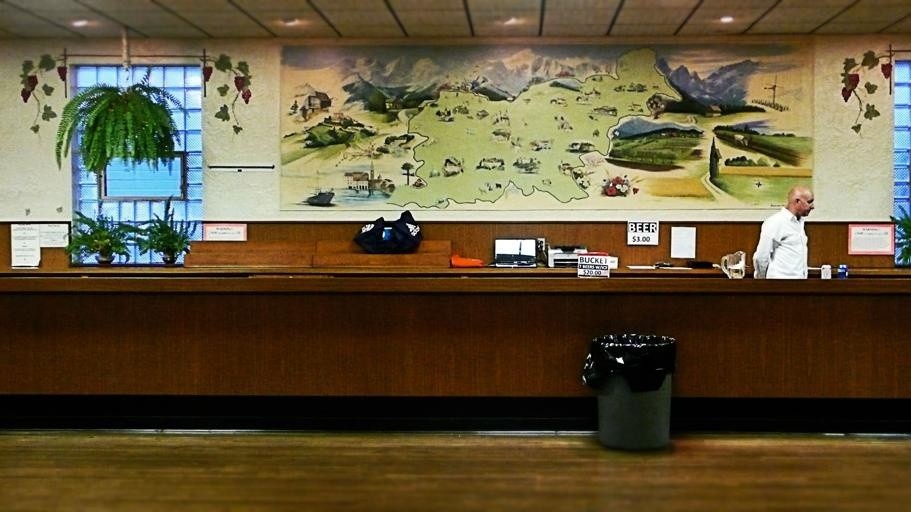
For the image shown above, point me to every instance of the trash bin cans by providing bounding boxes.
[594,334,675,452]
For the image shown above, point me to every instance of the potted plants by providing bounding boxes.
[61,195,190,268]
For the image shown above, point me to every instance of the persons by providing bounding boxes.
[753,184,815,279]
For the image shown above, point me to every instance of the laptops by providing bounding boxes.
[493,237,539,268]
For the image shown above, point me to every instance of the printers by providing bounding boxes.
[548,246,587,269]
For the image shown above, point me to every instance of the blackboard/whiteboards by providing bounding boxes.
[848,224,896,258]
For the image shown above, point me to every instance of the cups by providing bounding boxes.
[721,251,747,279]
